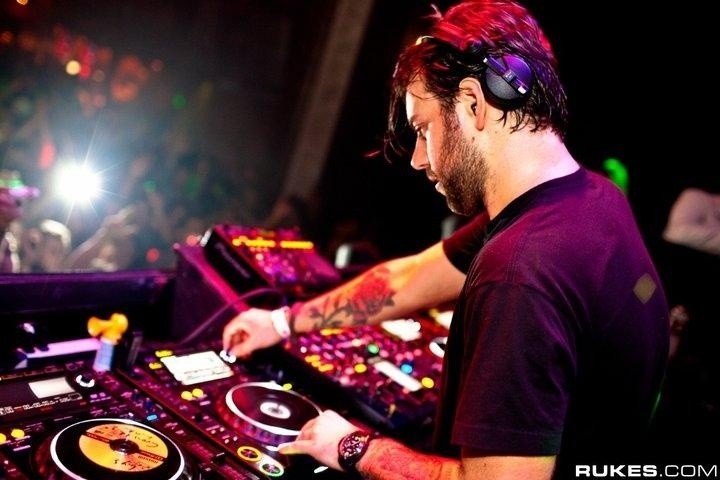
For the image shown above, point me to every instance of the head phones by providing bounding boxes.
[415,28,533,111]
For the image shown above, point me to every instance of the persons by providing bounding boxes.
[644,165,718,437]
[219,1,674,480]
[1,29,293,275]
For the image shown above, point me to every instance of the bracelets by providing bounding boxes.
[270,306,292,339]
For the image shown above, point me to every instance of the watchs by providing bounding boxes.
[336,428,382,480]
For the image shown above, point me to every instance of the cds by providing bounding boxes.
[79,423,168,473]
